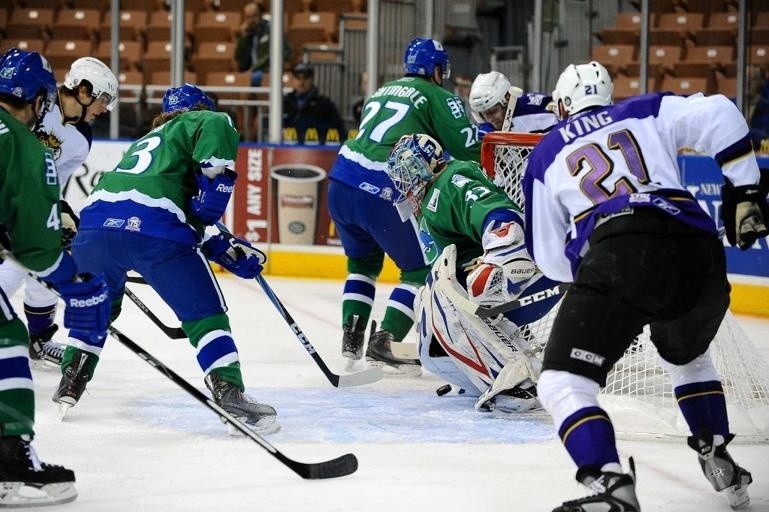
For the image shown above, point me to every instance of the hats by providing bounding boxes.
[292,64,315,80]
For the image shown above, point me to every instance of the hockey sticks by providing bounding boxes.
[122,285,187,340]
[217,221,384,388]
[29,272,358,479]
[443,230,726,319]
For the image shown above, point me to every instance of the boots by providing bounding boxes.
[687,433,752,491]
[495,378,541,410]
[1,435,75,482]
[342,313,364,360]
[51,352,94,403]
[366,320,421,368]
[29,325,65,364]
[552,457,640,512]
[203,372,277,425]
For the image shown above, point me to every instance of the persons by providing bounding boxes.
[325,39,495,372]
[468,70,561,170]
[232,3,290,81]
[388,133,568,421]
[0,45,111,485]
[352,73,367,130]
[728,65,769,229]
[280,63,348,145]
[521,61,769,512]
[0,55,120,365]
[52,81,278,427]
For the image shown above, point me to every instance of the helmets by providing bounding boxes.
[0,48,57,112]
[66,57,117,111]
[403,37,449,79]
[552,61,612,116]
[468,70,512,126]
[387,133,448,199]
[162,85,213,113]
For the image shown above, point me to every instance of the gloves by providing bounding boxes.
[719,170,768,249]
[64,272,112,334]
[192,170,234,226]
[201,233,266,280]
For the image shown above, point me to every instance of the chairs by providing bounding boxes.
[1,1,370,140]
[581,1,769,122]
